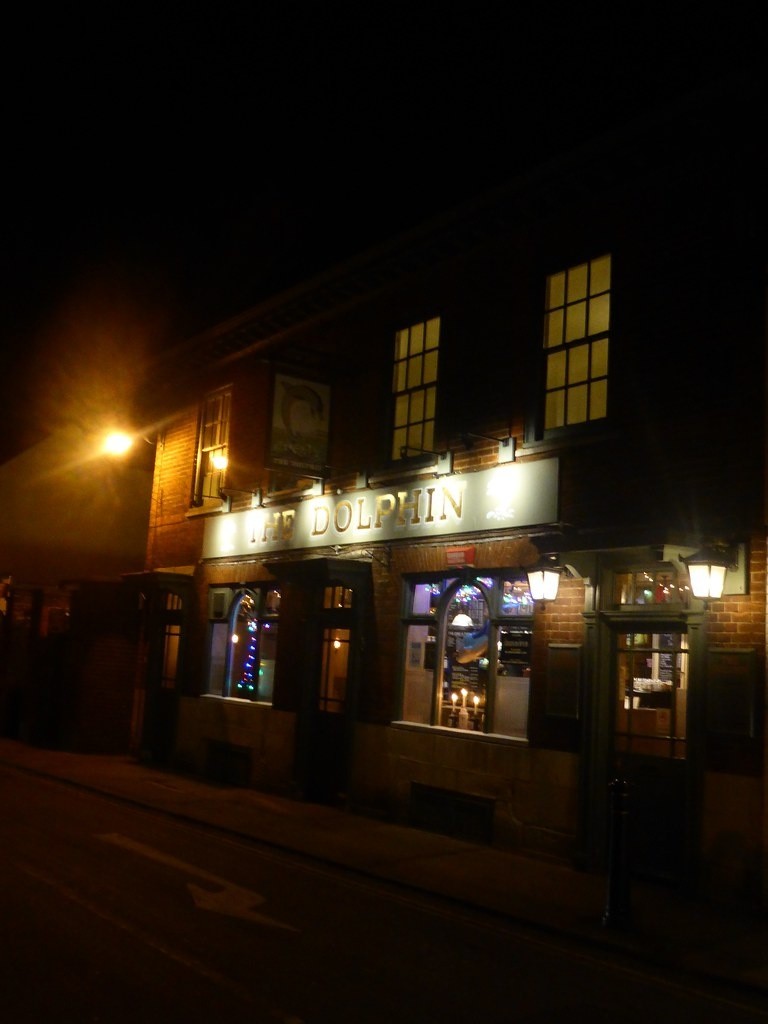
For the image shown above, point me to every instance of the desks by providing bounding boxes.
[623,690,676,713]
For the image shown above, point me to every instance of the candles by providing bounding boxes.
[451,687,482,715]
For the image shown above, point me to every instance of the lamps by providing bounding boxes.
[678,536,740,600]
[523,530,576,603]
